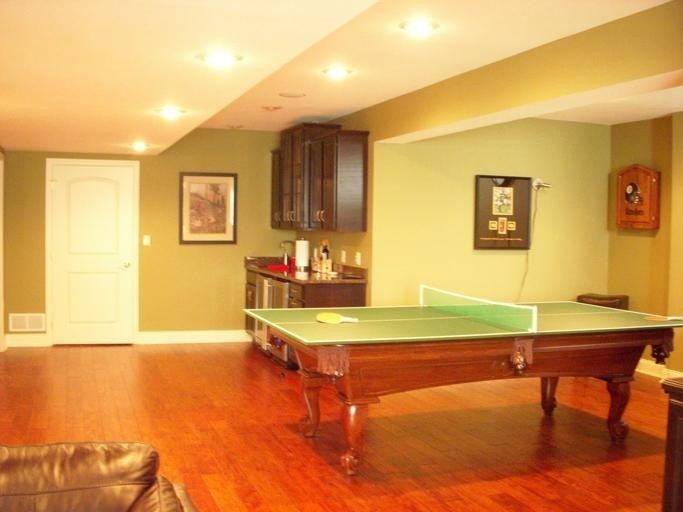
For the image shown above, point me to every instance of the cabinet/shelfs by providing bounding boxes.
[269,122,371,234]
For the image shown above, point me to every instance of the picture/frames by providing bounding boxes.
[179,171,237,245]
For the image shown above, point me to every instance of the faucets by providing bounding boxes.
[279,237,304,247]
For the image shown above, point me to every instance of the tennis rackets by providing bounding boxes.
[316,312,360,326]
[645,314,682,322]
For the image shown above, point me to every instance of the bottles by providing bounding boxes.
[322,244,329,261]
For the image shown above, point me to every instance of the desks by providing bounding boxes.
[241,284,683,478]
